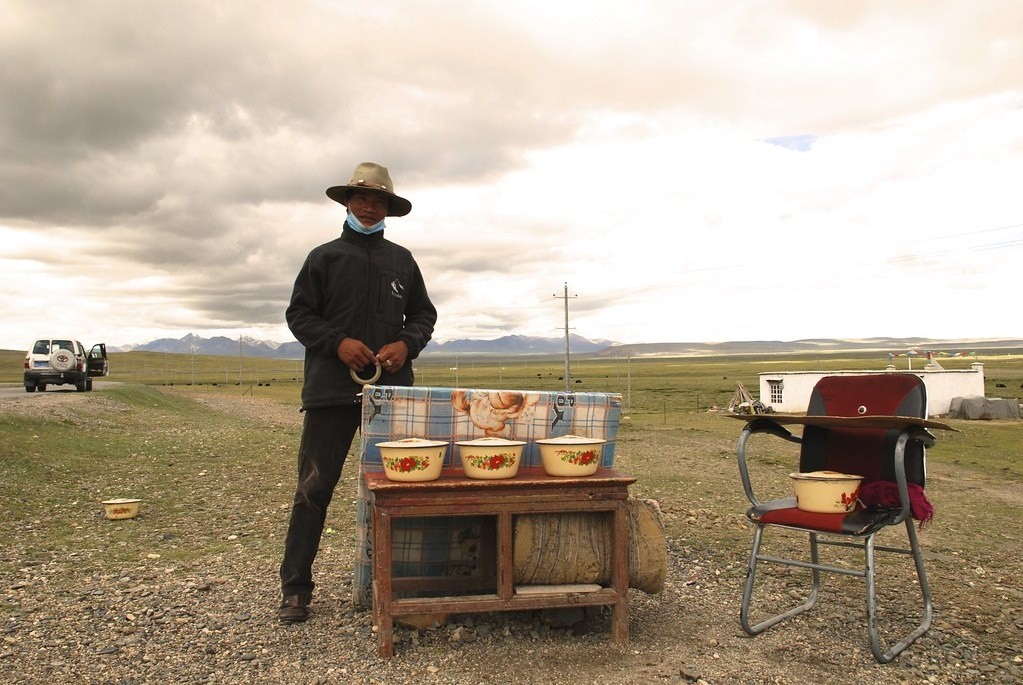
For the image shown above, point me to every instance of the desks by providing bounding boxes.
[358,467,638,656]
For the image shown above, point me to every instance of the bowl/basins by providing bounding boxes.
[789,470,865,513]
[375,437,450,481]
[100,498,142,520]
[534,435,607,476]
[453,435,527,482]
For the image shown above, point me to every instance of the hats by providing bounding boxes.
[326,161,412,218]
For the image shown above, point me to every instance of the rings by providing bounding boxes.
[386,359,394,366]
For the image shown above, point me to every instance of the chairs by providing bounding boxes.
[734,372,941,663]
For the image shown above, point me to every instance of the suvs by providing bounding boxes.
[22,337,110,393]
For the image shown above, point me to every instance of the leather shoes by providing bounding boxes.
[278,594,310,622]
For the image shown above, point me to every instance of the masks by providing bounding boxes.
[346,199,386,235]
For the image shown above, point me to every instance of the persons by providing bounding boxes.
[275,160,438,625]
